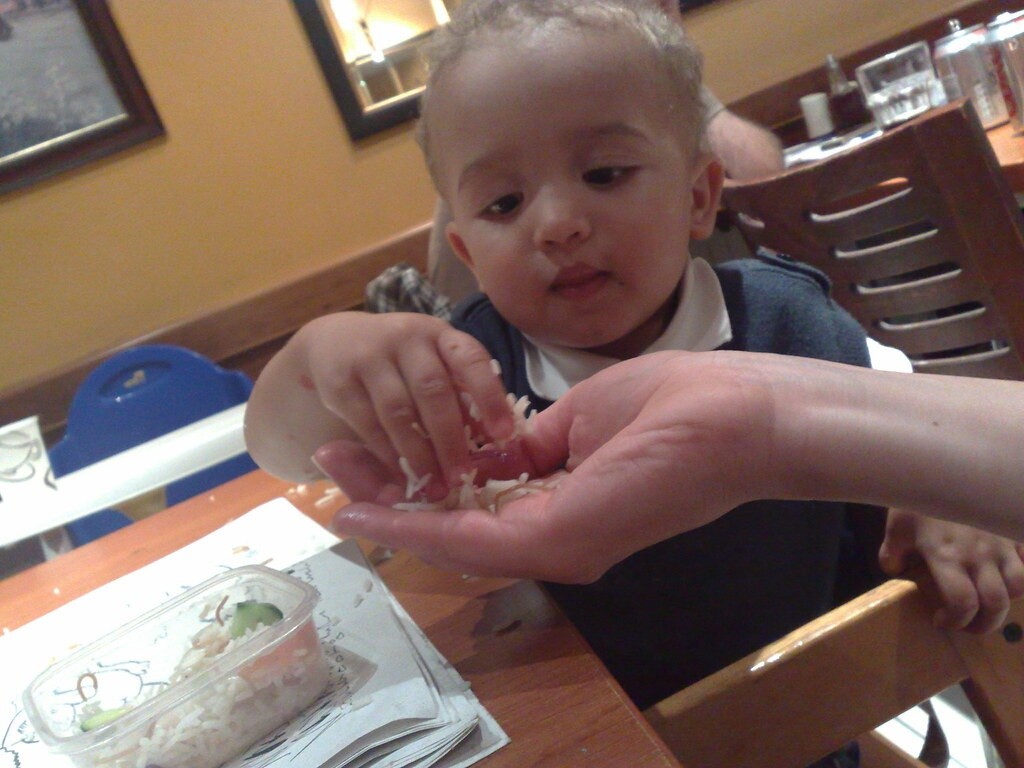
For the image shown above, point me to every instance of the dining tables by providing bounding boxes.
[0,457,680,768]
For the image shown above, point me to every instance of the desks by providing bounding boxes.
[782,113,1024,217]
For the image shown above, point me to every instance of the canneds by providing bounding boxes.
[986,8,1024,134]
[933,19,1010,130]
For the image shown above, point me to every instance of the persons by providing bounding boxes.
[245,0,1024,768]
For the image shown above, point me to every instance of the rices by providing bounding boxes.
[81,612,330,768]
[389,358,557,514]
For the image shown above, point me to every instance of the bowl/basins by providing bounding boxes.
[20,562,329,768]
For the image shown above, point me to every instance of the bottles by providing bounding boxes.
[825,54,868,134]
[799,93,835,142]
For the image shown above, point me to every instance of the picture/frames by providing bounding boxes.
[0,1,168,205]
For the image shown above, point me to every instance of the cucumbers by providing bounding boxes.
[80,707,133,732]
[230,600,283,637]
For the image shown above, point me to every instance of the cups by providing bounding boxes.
[0,415,57,505]
[854,40,946,133]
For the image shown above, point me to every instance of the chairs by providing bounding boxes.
[45,344,261,551]
[639,570,1024,767]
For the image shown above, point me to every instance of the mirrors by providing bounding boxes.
[292,0,714,144]
[720,99,1024,381]
